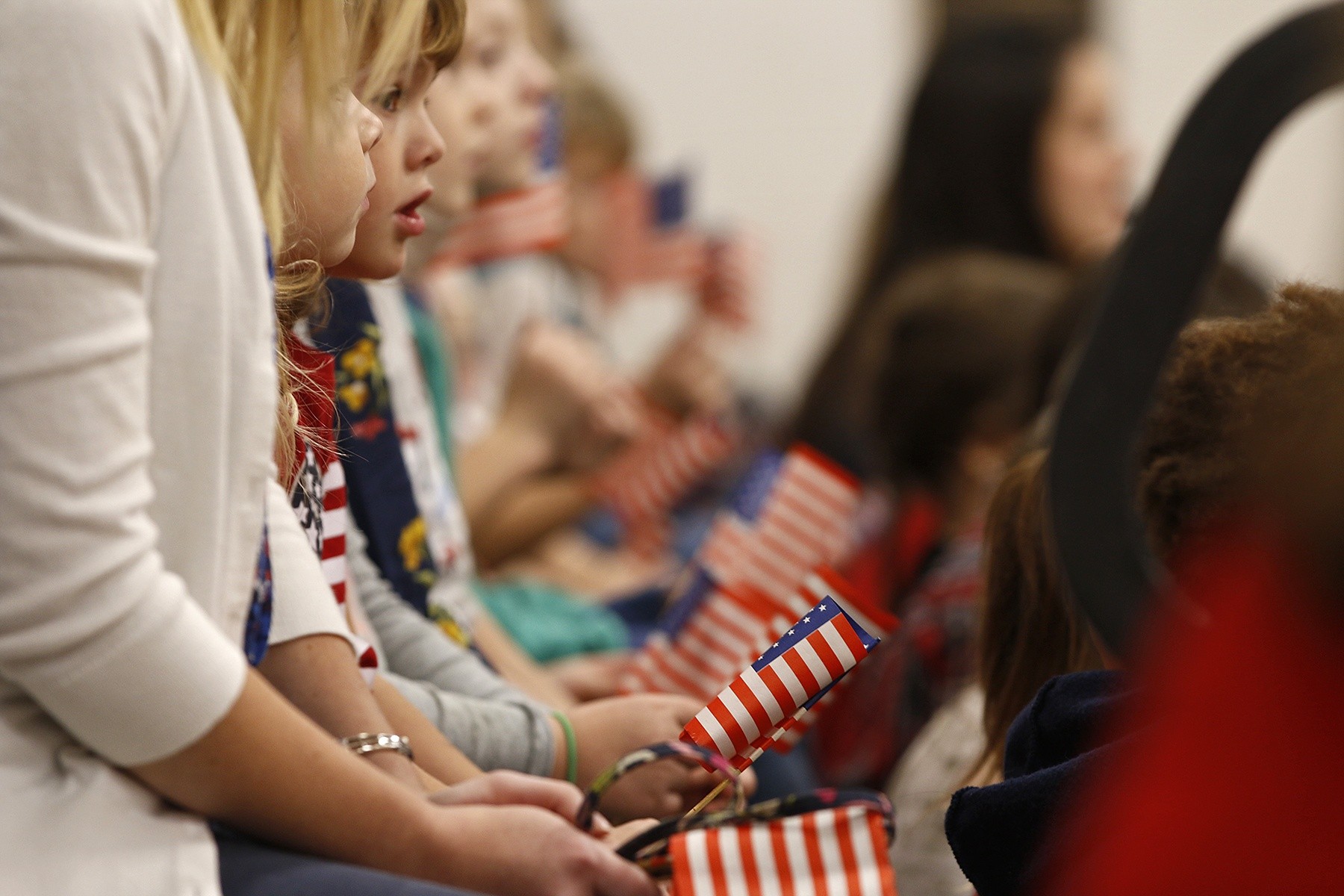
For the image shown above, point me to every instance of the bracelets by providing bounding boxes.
[344,732,413,757]
[550,709,577,783]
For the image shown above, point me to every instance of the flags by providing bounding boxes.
[486,174,899,896]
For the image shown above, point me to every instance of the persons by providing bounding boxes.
[1,0,755,896]
[801,2,1344,896]
